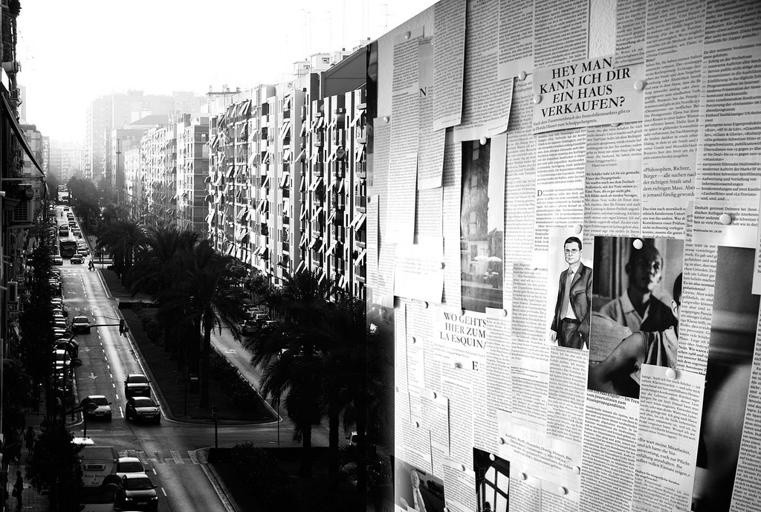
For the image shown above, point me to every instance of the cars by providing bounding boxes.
[345,431,367,448]
[49,207,90,400]
[239,307,289,360]
[78,445,158,511]
[85,394,112,422]
[124,373,161,425]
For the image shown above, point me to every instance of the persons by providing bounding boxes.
[605,243,669,329]
[396,470,441,512]
[588,271,681,396]
[550,237,593,350]
